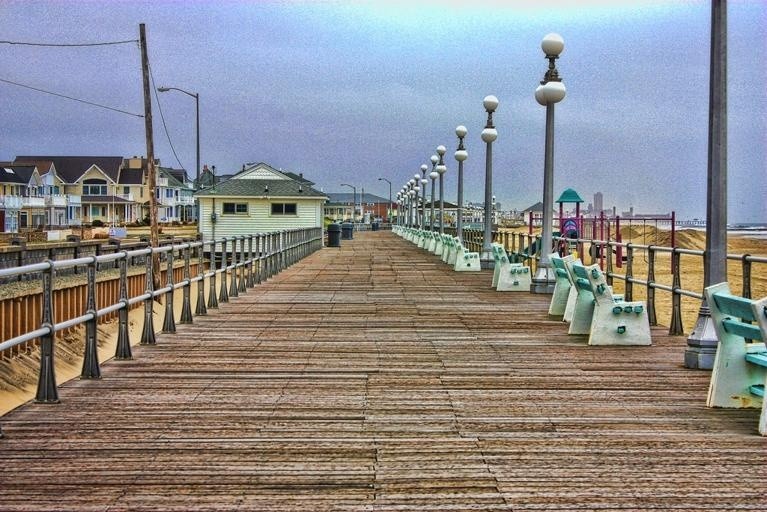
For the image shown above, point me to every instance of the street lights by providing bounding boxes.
[530,33,566,294]
[397,95,499,268]
[379,178,391,222]
[158,87,200,191]
[340,184,355,223]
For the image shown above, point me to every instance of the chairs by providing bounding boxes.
[548,251,652,346]
[441,234,481,271]
[704,281,765,435]
[490,242,531,291]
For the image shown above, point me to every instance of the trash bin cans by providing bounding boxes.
[327,224,341,247]
[372,222,378,231]
[341,223,353,240]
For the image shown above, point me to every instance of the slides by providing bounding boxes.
[507,231,560,264]
